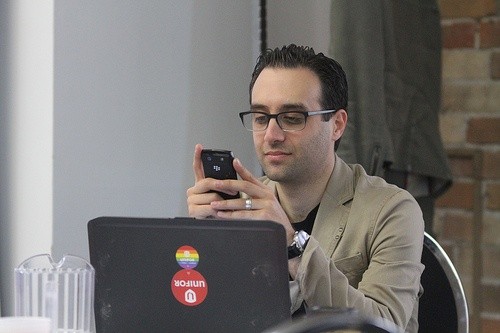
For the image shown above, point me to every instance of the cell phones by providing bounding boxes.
[201,149,241,200]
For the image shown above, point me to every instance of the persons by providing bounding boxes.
[186,43,425,333]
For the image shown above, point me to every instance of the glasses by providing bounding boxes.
[238,109,337,132]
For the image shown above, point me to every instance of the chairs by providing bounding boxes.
[418,231,470,333]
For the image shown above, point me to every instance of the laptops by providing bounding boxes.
[88,216,291,333]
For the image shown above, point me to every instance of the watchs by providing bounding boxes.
[288,230,310,260]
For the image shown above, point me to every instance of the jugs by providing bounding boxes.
[13,255,97,333]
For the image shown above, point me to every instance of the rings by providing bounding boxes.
[245,198,253,211]
